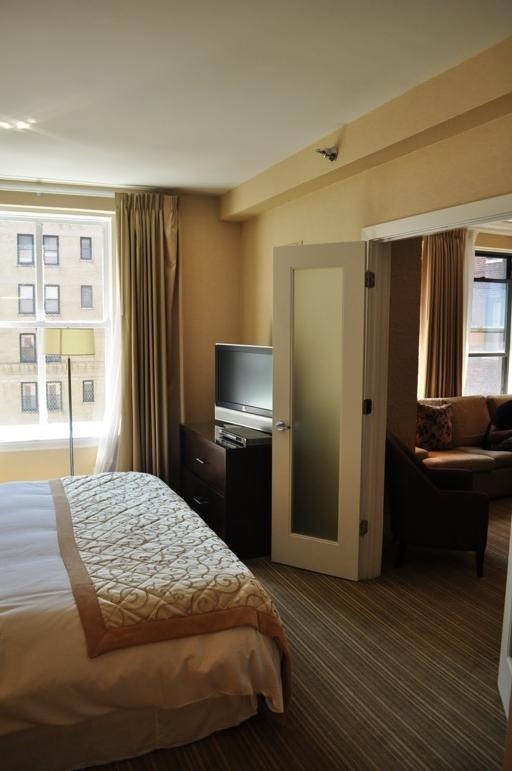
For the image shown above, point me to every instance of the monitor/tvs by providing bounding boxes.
[214,342,272,433]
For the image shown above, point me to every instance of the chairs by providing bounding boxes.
[386,430,491,577]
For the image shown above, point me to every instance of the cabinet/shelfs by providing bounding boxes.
[179,422,272,560]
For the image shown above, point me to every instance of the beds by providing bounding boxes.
[0,471,285,770]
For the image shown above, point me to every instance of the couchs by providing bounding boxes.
[416,396,512,499]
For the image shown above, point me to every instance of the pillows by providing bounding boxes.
[416,399,458,449]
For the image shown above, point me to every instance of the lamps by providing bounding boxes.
[43,327,96,476]
